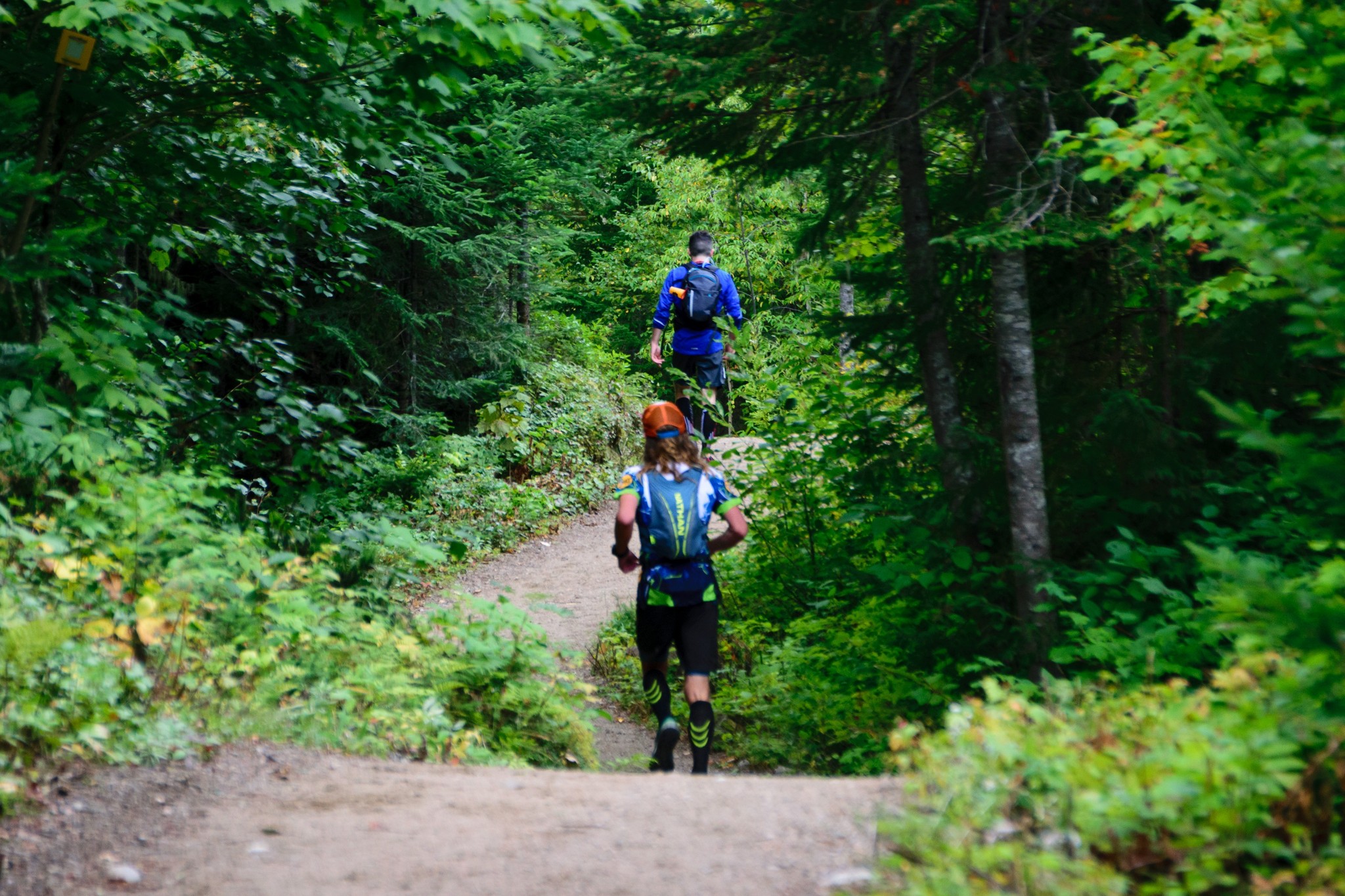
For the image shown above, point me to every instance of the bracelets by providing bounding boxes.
[612,544,629,558]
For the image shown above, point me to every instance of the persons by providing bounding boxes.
[612,401,748,775]
[650,230,743,448]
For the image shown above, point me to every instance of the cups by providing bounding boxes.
[669,286,686,299]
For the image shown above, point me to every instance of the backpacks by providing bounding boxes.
[678,263,722,328]
[637,467,708,561]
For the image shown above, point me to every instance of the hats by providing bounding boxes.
[641,400,686,439]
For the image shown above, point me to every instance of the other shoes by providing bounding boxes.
[648,718,680,771]
[701,456,716,467]
